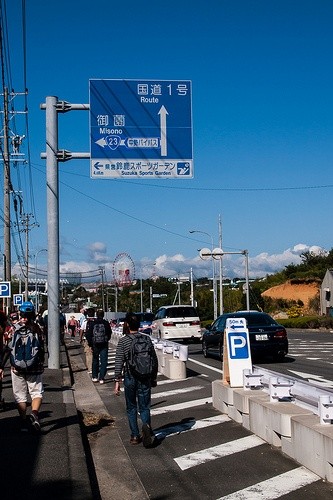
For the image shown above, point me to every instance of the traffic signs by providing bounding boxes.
[88,78,195,180]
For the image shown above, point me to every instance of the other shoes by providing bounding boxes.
[92,377,97,383]
[142,424,152,448]
[28,413,40,432]
[99,379,104,384]
[20,425,28,431]
[130,436,138,445]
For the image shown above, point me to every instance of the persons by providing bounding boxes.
[109,319,113,327]
[0,310,65,411]
[0,299,45,432]
[68,316,76,341]
[88,310,112,383]
[122,312,135,336]
[78,308,96,373]
[114,315,158,448]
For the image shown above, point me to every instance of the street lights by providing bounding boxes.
[189,230,217,320]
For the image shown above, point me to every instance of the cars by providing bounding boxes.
[130,311,154,334]
[150,304,201,344]
[201,310,289,364]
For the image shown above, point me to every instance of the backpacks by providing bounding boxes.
[92,320,107,345]
[125,334,154,381]
[9,322,43,375]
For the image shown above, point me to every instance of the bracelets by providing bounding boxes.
[114,380,119,383]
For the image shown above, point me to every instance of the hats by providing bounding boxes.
[19,301,33,312]
